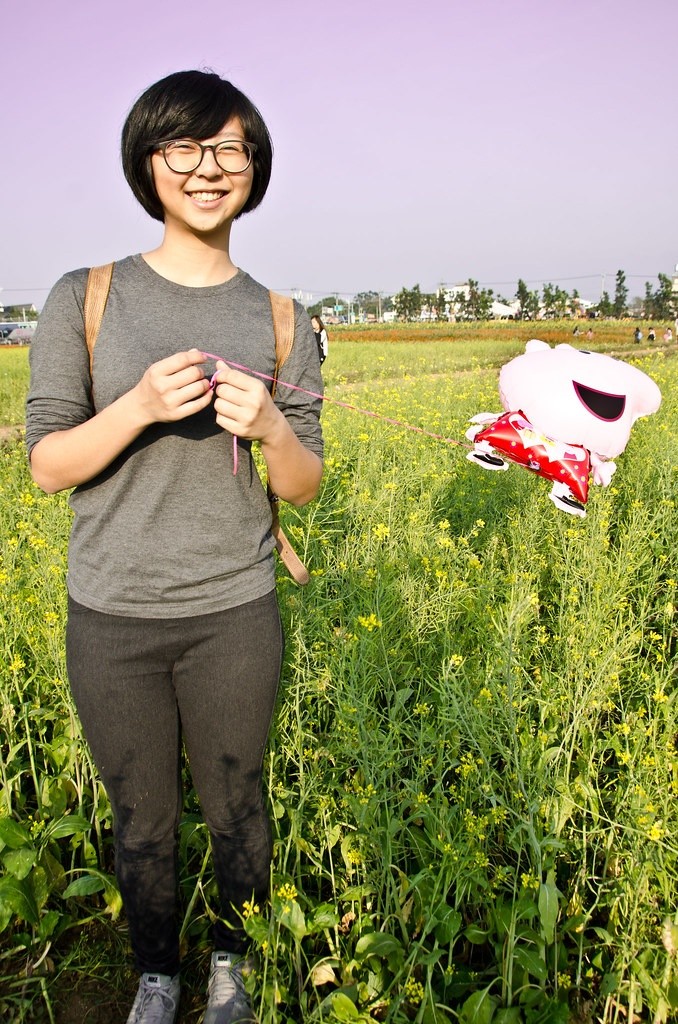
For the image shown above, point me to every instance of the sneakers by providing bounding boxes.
[127,970,181,1024]
[205,951,258,1024]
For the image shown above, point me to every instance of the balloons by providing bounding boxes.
[466,339,661,518]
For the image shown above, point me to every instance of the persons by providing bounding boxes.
[573,327,584,337]
[586,328,593,340]
[311,315,328,367]
[664,329,673,340]
[648,327,655,341]
[634,327,642,343]
[24,72,325,1024]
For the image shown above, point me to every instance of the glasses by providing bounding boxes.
[150,138,259,174]
